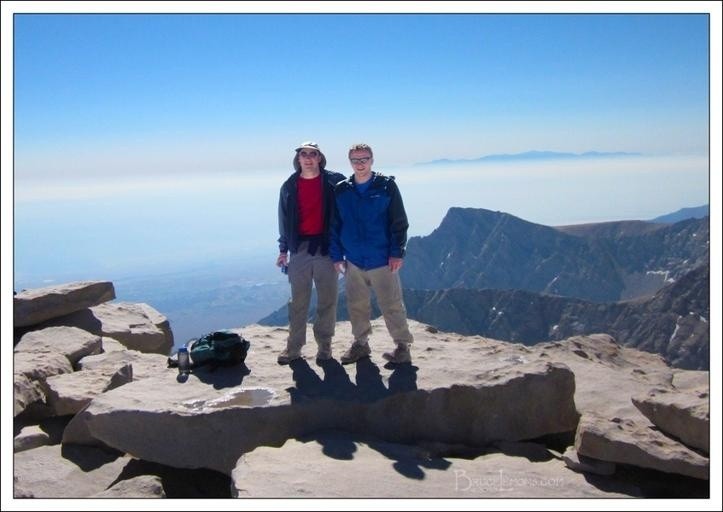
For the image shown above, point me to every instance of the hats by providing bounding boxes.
[295,141,319,152]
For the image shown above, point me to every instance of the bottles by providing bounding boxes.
[178,347,190,376]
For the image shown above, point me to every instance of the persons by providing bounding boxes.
[276,141,384,369]
[328,143,414,365]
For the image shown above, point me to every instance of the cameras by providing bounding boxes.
[281,263,288,274]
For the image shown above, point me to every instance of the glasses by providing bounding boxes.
[299,151,316,158]
[350,157,371,164]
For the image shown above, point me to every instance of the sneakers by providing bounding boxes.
[316,342,332,361]
[340,340,372,363]
[382,344,412,364]
[277,348,301,363]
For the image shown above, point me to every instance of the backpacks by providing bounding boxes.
[189,329,251,368]
